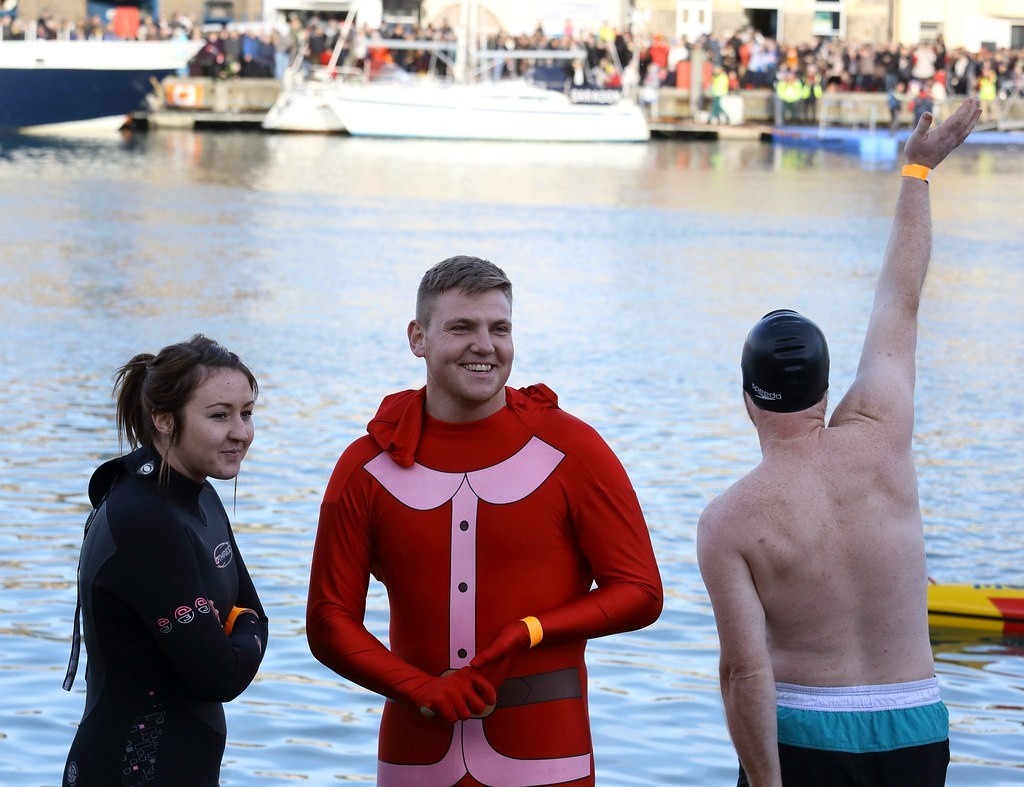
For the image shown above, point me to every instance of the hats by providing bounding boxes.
[741,308,830,413]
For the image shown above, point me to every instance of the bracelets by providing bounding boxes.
[223,606,258,636]
[899,161,934,183]
[517,616,543,648]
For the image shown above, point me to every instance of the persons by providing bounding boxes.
[59,335,271,786]
[697,82,984,786]
[306,253,667,787]
[2,2,1019,133]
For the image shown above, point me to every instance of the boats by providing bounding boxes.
[262,1,650,141]
[0,0,206,133]
[924,583,1024,646]
